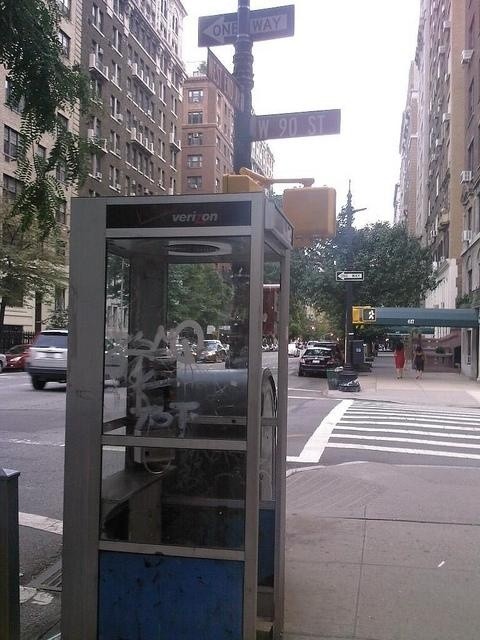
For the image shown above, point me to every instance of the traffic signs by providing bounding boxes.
[253,110,342,140]
[198,4,295,47]
[336,271,364,281]
[204,47,246,116]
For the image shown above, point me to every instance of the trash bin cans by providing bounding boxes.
[159,368,279,501]
[326,368,342,390]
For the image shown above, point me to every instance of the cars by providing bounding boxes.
[0,354,8,372]
[4,343,32,371]
[262,344,278,352]
[193,344,197,351]
[223,344,230,352]
[194,340,228,362]
[174,344,193,359]
[288,343,300,357]
[299,347,338,377]
[296,342,304,350]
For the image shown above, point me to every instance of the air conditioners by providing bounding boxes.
[95,171,103,180]
[458,48,474,64]
[460,170,473,183]
[426,1,453,247]
[116,113,123,123]
[461,229,472,243]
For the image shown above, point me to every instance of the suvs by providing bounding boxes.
[306,341,318,349]
[29,329,129,391]
[314,342,342,368]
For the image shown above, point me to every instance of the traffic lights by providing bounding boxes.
[359,308,376,322]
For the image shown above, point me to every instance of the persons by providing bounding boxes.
[384,342,388,351]
[414,344,426,379]
[394,341,407,379]
